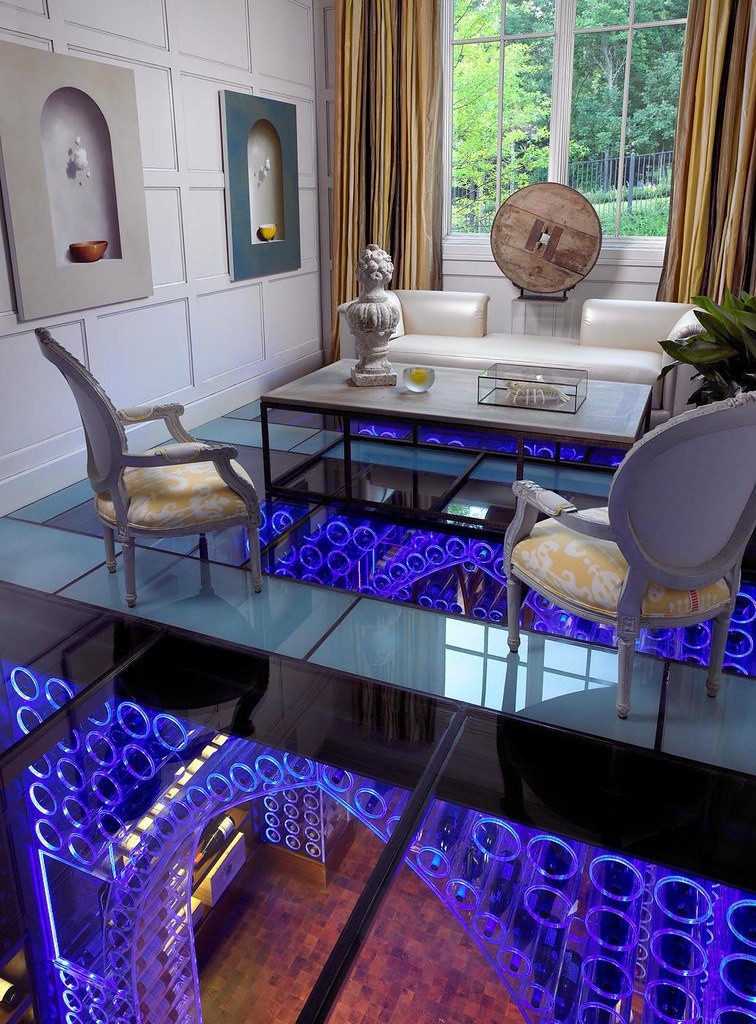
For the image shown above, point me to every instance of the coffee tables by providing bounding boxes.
[260,359,653,542]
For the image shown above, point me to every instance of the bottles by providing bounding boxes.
[430,803,467,871]
[664,882,695,1014]
[0,978,24,1012]
[456,819,498,902]
[484,851,521,938]
[593,861,631,1024]
[540,871,569,918]
[24,671,283,1024]
[510,887,543,971]
[742,907,756,995]
[553,949,582,1024]
[332,769,345,784]
[532,915,565,1008]
[287,786,338,854]
[240,502,748,678]
[294,756,307,772]
[546,833,567,875]
[365,781,393,813]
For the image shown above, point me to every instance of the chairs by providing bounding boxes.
[503,388,756,721]
[33,327,263,609]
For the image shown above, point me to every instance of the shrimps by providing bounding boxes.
[505,381,571,406]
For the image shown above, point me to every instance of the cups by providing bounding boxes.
[259,224,277,240]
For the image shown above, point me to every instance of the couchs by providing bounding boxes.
[335,289,709,429]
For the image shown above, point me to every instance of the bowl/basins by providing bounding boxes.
[69,241,108,261]
[403,367,434,392]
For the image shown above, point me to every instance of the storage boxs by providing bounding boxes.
[476,362,589,414]
